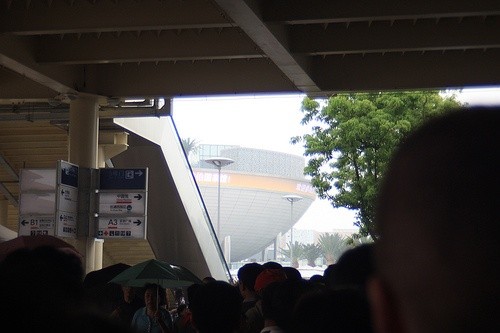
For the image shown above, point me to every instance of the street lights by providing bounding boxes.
[204,157,235,239]
[282,194,304,264]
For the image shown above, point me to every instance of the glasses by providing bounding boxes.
[144,295,160,300]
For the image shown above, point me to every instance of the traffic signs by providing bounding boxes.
[56,160,82,239]
[98,167,148,241]
[16,215,56,238]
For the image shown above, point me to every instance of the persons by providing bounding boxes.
[0,236,379,333]
[367,104,500,333]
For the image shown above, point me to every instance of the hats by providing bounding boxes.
[256,269,287,291]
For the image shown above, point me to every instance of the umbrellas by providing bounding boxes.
[111,261,202,314]
